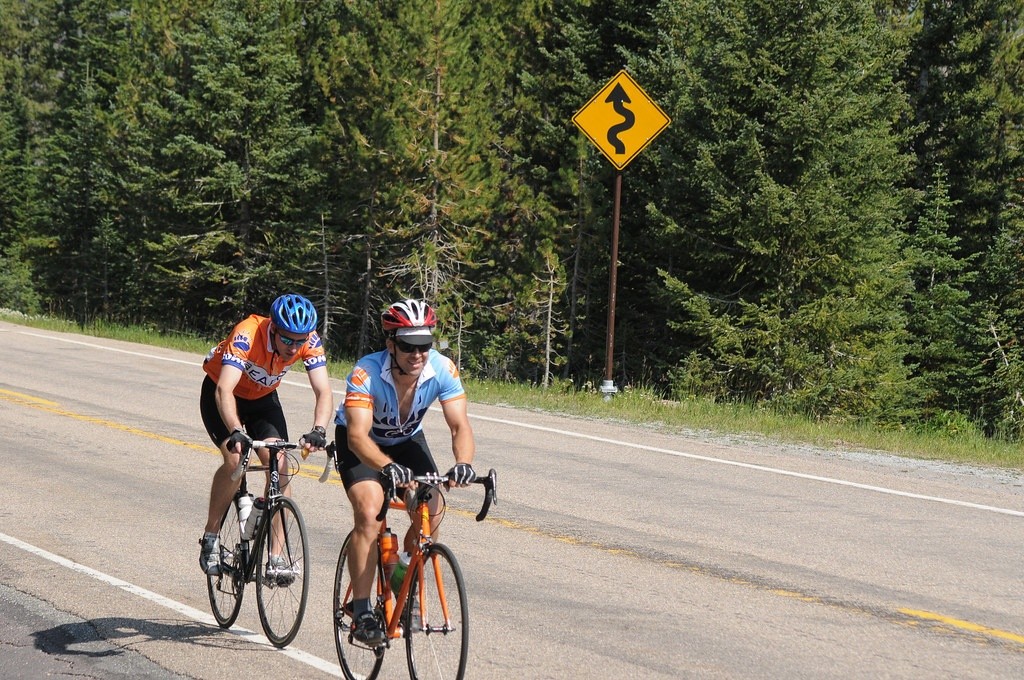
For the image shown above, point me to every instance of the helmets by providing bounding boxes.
[381,299,438,345]
[270,294,318,334]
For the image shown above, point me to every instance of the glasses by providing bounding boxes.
[276,331,309,347]
[391,337,433,354]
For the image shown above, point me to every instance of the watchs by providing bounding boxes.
[312,426,326,437]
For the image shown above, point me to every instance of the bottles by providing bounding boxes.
[237,493,253,533]
[391,552,411,593]
[382,528,400,581]
[245,497,265,536]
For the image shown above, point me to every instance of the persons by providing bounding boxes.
[334,298,477,644]
[199,294,333,585]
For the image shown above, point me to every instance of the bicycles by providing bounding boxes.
[330,468,499,680]
[205,434,337,648]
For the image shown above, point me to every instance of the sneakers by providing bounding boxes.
[349,609,385,648]
[265,557,296,588]
[198,532,223,575]
[394,590,421,634]
